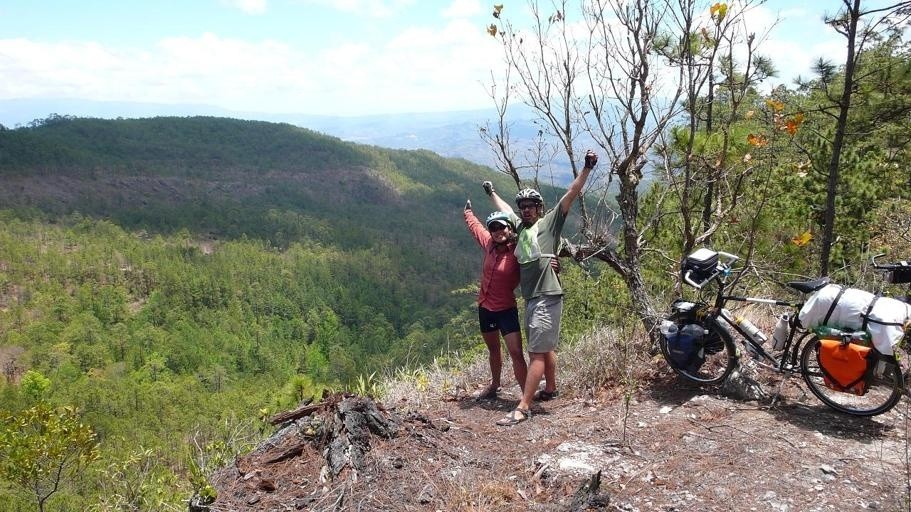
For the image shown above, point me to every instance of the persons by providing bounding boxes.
[482,150,597,426]
[463,199,561,398]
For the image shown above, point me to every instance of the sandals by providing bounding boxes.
[534,389,560,400]
[496,407,532,426]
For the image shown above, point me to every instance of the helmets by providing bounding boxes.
[486,212,515,231]
[514,188,543,205]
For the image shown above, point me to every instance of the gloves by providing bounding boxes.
[465,202,472,210]
[555,259,562,274]
[584,149,598,169]
[482,181,496,196]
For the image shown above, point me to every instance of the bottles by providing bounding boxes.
[808,325,872,346]
[735,312,789,363]
[681,324,709,336]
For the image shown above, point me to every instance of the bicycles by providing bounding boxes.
[871,252,910,350]
[659,247,904,417]
